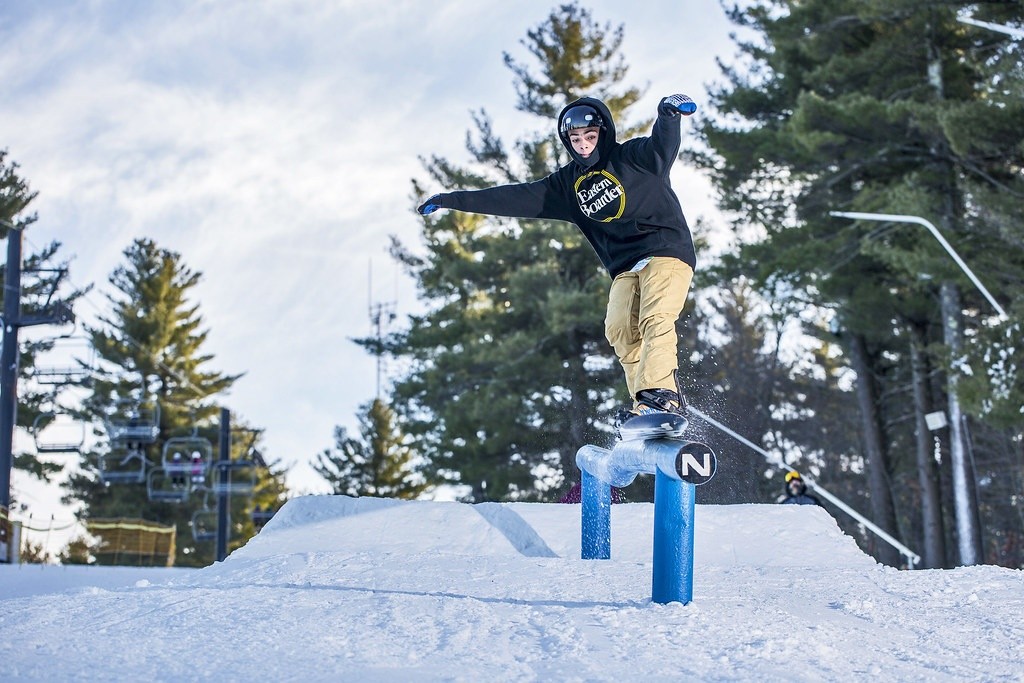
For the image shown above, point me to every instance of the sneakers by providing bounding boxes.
[586,390,685,432]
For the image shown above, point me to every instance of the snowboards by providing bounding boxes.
[618,411,689,441]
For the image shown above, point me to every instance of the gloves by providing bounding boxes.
[417,193,442,214]
[663,94,697,118]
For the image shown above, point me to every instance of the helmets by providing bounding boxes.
[560,105,605,137]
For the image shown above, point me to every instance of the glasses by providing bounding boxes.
[783,471,799,482]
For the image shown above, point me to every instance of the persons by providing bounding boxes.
[559,478,622,505]
[418,94,697,432]
[776,471,820,505]
[171,453,185,488]
[189,451,205,494]
